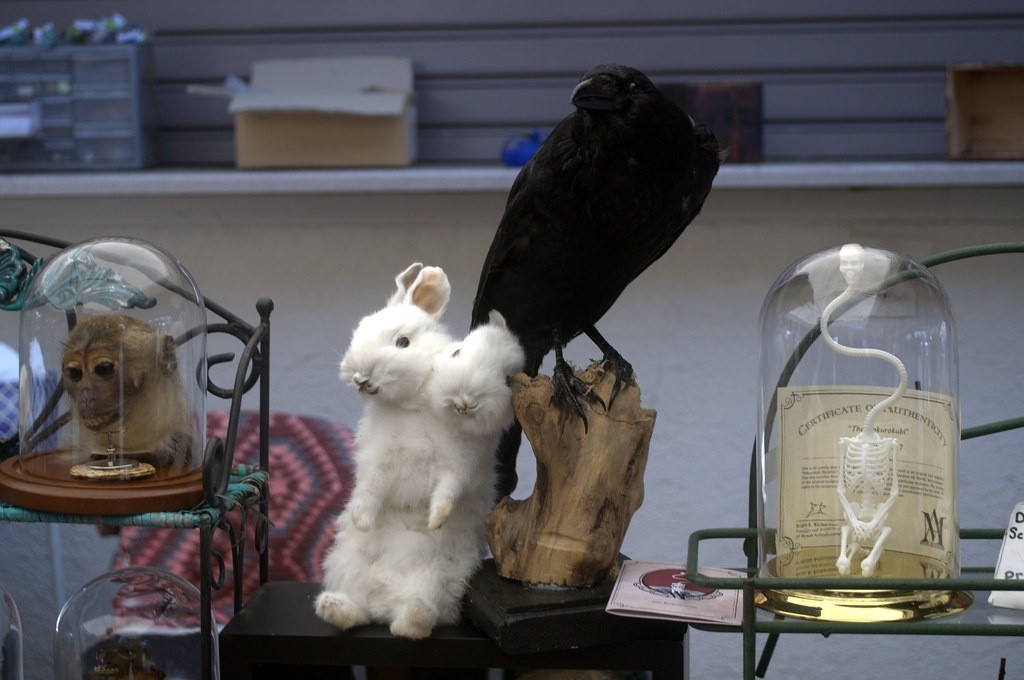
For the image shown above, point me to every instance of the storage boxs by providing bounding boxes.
[0,42,149,171]
[228,57,419,169]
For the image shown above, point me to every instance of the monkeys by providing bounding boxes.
[58,313,202,478]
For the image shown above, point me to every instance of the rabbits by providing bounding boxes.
[313,262,527,639]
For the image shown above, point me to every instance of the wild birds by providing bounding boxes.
[469,64,723,509]
